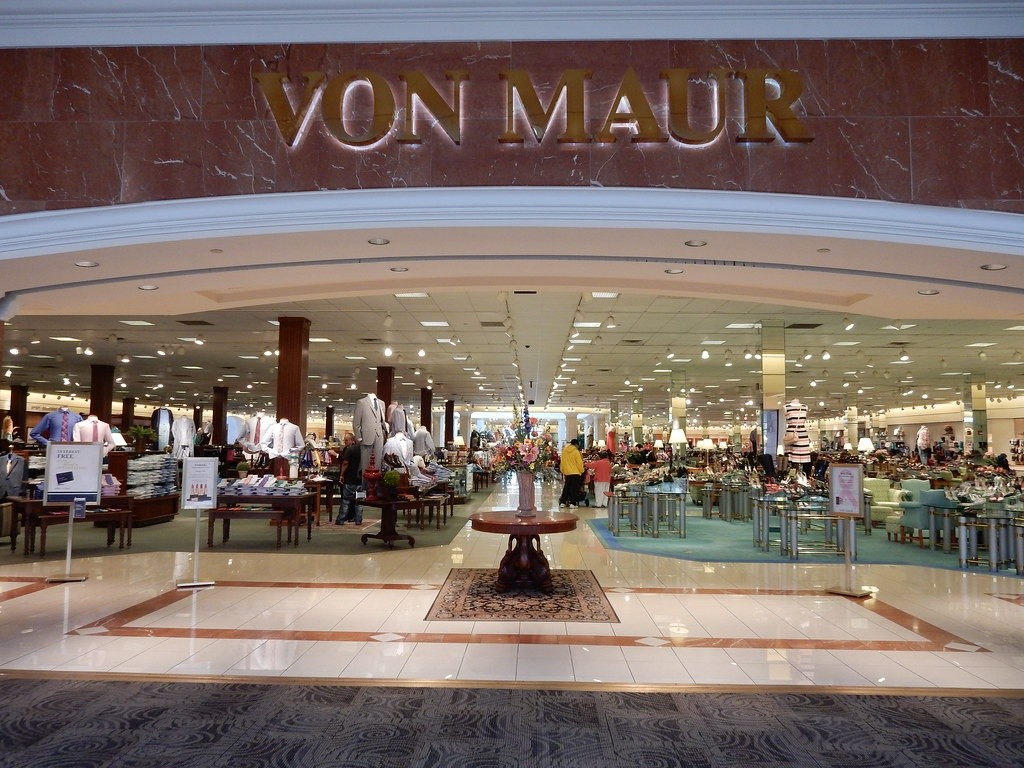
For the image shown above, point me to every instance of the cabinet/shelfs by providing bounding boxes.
[21,450,46,499]
[94,449,178,528]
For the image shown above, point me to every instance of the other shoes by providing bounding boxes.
[348,518,355,522]
[335,514,350,525]
[592,505,601,508]
[355,519,362,525]
[647,467,687,486]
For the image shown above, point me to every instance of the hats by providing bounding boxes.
[566,439,579,444]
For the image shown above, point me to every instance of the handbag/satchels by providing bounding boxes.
[783,431,798,446]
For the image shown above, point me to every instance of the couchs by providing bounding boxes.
[900,478,931,491]
[863,478,905,528]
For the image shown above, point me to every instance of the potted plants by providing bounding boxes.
[383,471,400,501]
[129,425,156,451]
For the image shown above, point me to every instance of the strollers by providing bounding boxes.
[566,467,590,508]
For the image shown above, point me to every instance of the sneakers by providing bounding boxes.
[568,503,578,509]
[559,503,566,508]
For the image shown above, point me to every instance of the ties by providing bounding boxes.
[373,398,378,412]
[6,459,11,474]
[278,423,285,453]
[61,411,69,442]
[254,417,261,446]
[93,421,98,443]
[403,409,408,433]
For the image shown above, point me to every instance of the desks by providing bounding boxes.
[357,499,420,548]
[923,498,1024,576]
[205,475,334,549]
[469,511,579,595]
[404,485,456,530]
[474,471,489,492]
[605,471,872,560]
[7,495,133,556]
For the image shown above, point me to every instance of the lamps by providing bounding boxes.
[697,441,704,448]
[719,441,727,448]
[669,429,687,444]
[700,438,713,467]
[606,316,1024,424]
[5,290,527,415]
[857,438,875,465]
[654,439,664,451]
[544,311,605,412]
[844,442,852,452]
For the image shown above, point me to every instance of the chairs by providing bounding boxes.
[900,490,958,548]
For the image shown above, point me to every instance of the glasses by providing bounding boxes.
[343,439,349,442]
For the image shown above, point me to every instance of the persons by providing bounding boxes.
[607,424,616,453]
[29,408,83,445]
[384,400,414,465]
[413,426,435,462]
[587,425,593,448]
[353,394,389,471]
[559,439,585,509]
[73,415,116,457]
[584,451,612,508]
[917,425,929,465]
[151,407,211,457]
[228,413,274,451]
[1,415,13,441]
[750,428,756,460]
[635,444,657,462]
[783,400,811,479]
[260,418,305,461]
[327,433,363,525]
[983,452,1010,469]
[933,441,938,452]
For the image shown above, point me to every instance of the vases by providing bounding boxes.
[517,468,540,517]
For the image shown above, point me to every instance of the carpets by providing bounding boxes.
[423,568,621,623]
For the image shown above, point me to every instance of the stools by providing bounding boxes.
[886,516,914,543]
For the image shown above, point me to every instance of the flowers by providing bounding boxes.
[483,399,560,482]
